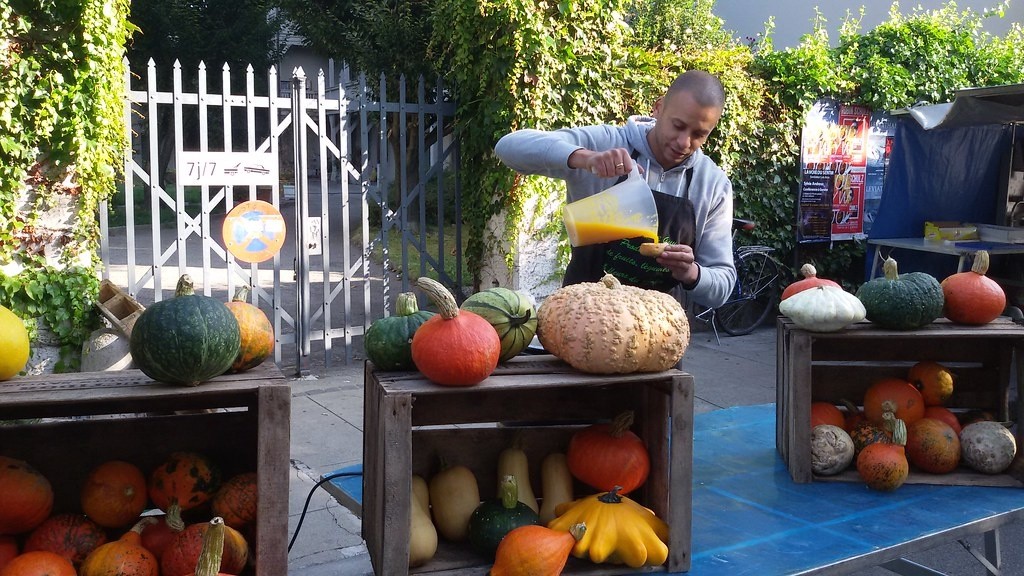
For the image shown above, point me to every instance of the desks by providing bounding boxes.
[320,399,1024,576]
[870,237,1024,283]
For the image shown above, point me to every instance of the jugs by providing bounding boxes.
[564,157,660,247]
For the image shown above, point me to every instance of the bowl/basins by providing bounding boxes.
[640,241,669,257]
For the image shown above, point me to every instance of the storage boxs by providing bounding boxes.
[925,221,979,241]
[361,353,696,576]
[979,224,1024,244]
[774,315,1024,487]
[0,361,292,576]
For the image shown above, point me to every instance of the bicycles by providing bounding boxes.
[666,215,793,347]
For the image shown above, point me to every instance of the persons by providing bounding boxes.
[490,70,741,311]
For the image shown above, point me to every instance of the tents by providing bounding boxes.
[862,84,1024,295]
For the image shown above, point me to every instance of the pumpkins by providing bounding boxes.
[0,249,1016,575]
